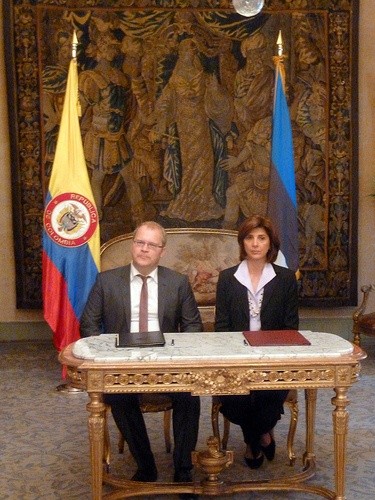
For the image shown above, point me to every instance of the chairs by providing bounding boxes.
[106,306,299,465]
[351,283,375,348]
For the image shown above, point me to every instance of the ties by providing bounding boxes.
[137,273,151,332]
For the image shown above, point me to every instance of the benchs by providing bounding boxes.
[100,228,242,322]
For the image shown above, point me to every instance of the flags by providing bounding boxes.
[266,56,300,281]
[44,59,103,379]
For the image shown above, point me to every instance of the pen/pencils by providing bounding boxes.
[171,339,174,345]
[243,339,248,346]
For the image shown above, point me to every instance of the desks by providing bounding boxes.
[59,331,368,500]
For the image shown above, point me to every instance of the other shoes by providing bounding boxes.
[245,443,265,468]
[260,431,276,462]
[131,469,159,482]
[171,452,193,483]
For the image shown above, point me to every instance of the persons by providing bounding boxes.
[79,222,204,486]
[214,215,298,470]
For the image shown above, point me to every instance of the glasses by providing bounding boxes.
[132,239,166,250]
